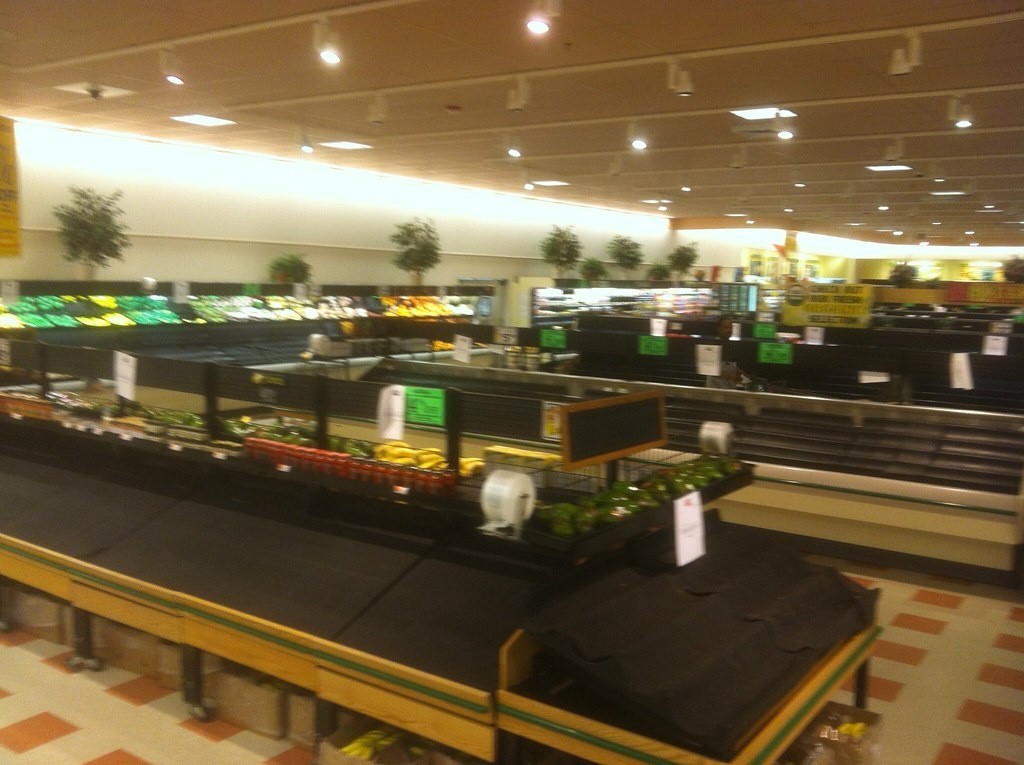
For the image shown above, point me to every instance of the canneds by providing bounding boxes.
[242,437,457,496]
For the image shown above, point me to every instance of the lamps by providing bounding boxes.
[368,91,389,127]
[667,61,695,99]
[159,45,185,86]
[947,93,975,129]
[313,16,341,65]
[774,108,798,141]
[888,31,924,76]
[505,75,532,112]
[884,137,906,164]
[526,0,563,33]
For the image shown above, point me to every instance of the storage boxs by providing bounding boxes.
[0,585,457,764]
